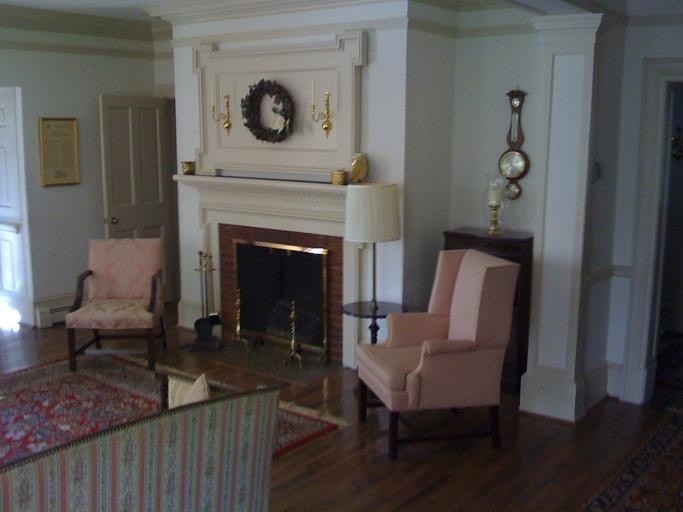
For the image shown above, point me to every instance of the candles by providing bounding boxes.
[209,84,217,108]
[311,78,316,109]
[198,224,209,255]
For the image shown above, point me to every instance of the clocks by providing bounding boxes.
[496,87,530,201]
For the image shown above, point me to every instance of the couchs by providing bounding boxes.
[353,244,525,462]
[60,233,170,374]
[0,368,283,510]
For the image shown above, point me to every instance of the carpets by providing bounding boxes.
[576,408,681,512]
[0,344,346,470]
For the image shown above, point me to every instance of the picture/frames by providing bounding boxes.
[37,114,80,188]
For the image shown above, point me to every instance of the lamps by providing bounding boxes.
[333,179,409,409]
[486,179,506,234]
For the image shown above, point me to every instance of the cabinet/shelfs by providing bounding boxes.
[437,221,531,396]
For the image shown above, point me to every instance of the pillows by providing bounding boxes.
[178,372,211,407]
